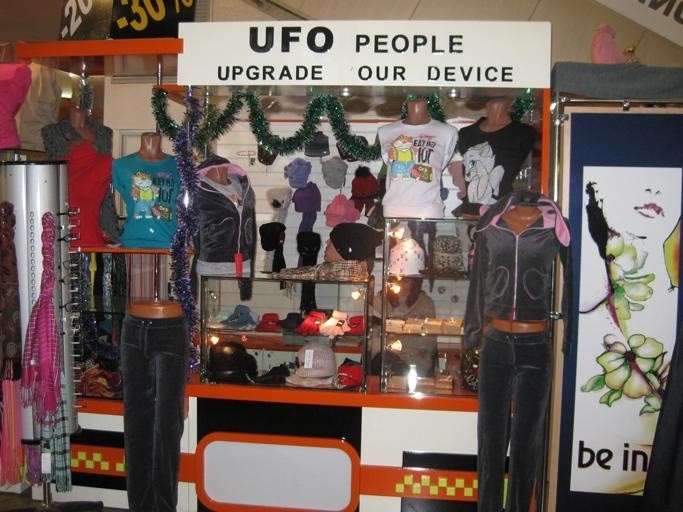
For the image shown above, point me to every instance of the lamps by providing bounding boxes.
[206,331,219,346]
[387,227,404,241]
[383,338,402,352]
[350,286,366,301]
[386,282,400,294]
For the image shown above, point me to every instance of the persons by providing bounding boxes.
[54,138,113,247]
[459,191,574,512]
[375,98,458,219]
[40,104,121,244]
[367,277,437,375]
[118,298,193,512]
[111,130,187,249]
[322,222,384,281]
[0,62,33,150]
[458,98,541,215]
[581,165,679,348]
[189,154,255,279]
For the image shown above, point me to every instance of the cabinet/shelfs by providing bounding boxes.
[381,214,483,396]
[199,271,374,395]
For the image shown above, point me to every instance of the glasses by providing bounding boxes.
[56,206,85,339]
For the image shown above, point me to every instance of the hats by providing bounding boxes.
[204,341,257,386]
[284,343,336,389]
[259,131,386,262]
[387,236,469,279]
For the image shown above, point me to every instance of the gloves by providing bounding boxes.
[206,304,364,341]
